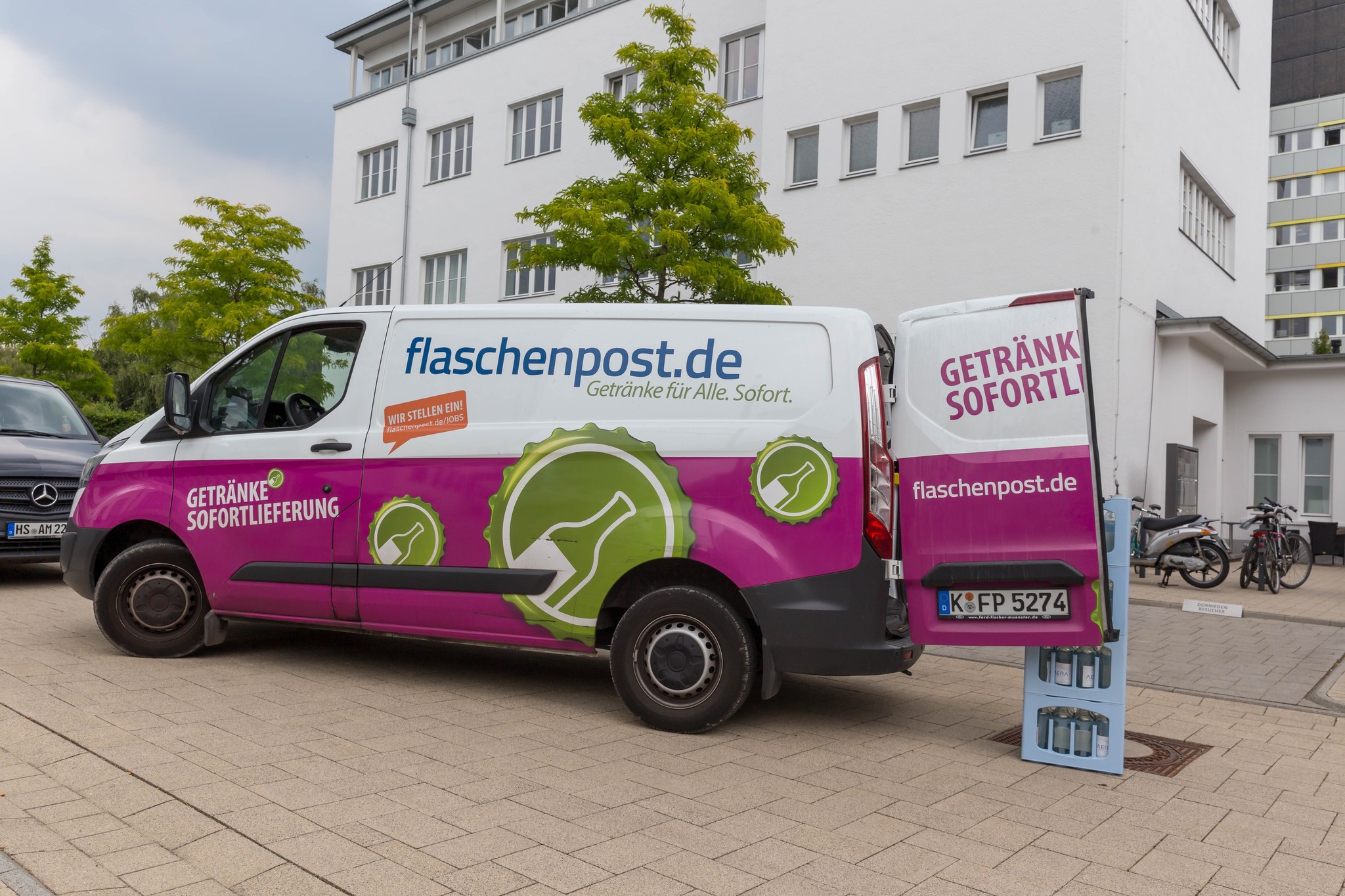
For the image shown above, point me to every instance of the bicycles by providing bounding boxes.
[1130,503,1162,573]
[1232,496,1313,594]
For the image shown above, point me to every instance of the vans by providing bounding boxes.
[56,282,1111,734]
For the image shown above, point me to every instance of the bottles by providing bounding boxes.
[62,416,71,434]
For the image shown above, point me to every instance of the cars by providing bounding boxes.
[0,374,110,568]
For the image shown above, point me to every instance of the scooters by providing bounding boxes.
[1104,478,1230,589]
